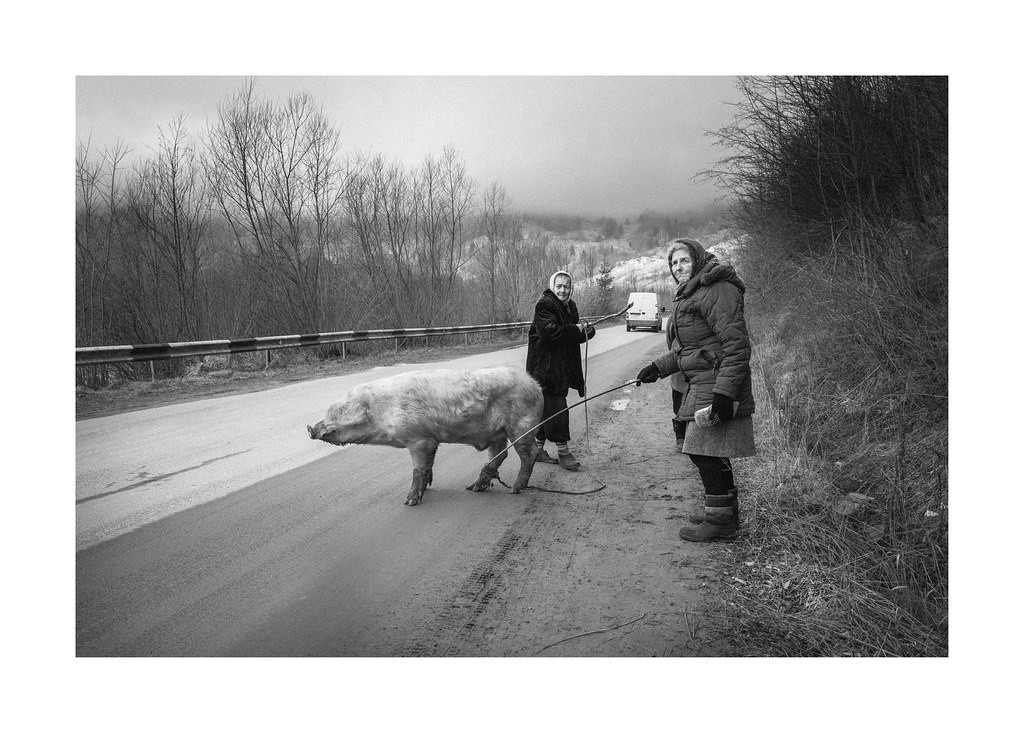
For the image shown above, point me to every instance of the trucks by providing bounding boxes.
[626,292,665,333]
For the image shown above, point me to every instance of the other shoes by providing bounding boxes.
[535,449,559,464]
[558,452,580,469]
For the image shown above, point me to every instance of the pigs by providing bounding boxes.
[307,366,545,506]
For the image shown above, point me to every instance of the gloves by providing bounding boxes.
[636,364,661,386]
[710,393,734,423]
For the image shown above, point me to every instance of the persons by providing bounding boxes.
[524,270,597,470]
[635,236,757,542]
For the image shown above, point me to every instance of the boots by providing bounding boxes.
[679,486,739,541]
